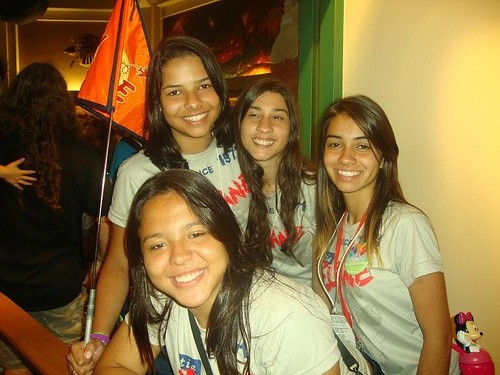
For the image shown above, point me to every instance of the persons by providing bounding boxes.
[0,62,460,375]
[75,35,252,375]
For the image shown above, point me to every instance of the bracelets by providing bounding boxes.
[91,334,110,343]
[101,340,107,347]
[92,331,110,337]
